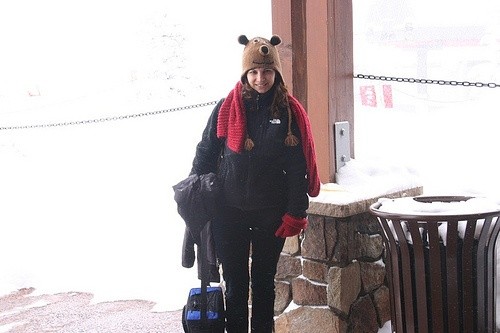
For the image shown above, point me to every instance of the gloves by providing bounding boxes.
[275,212,308,239]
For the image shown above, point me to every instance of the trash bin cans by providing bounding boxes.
[368,194,500,333]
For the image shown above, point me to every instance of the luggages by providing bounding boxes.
[182,226,225,333]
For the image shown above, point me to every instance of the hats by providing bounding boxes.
[238,34,300,151]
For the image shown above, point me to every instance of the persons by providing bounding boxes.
[185,36,309,333]
